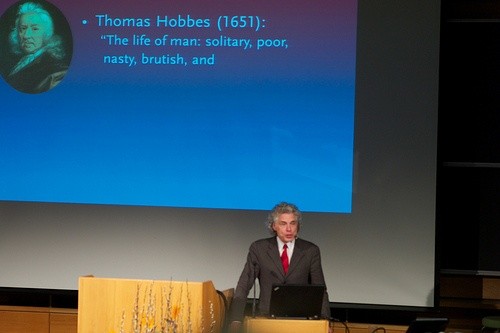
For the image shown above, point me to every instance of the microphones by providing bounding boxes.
[252,260,257,319]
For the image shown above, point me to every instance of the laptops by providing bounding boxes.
[268,283,325,318]
[406,317,448,333]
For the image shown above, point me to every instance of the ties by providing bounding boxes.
[280,244,289,274]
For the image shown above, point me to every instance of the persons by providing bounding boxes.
[227,202,327,333]
[5,1,71,94]
